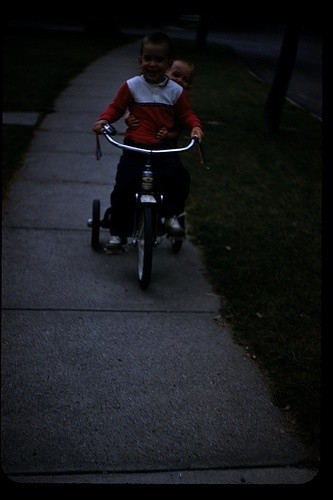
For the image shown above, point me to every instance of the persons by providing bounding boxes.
[123,54,194,141]
[93,33,204,245]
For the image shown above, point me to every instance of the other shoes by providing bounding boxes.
[106,235,123,251]
[163,216,183,232]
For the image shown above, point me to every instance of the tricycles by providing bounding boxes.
[89,123,200,287]
